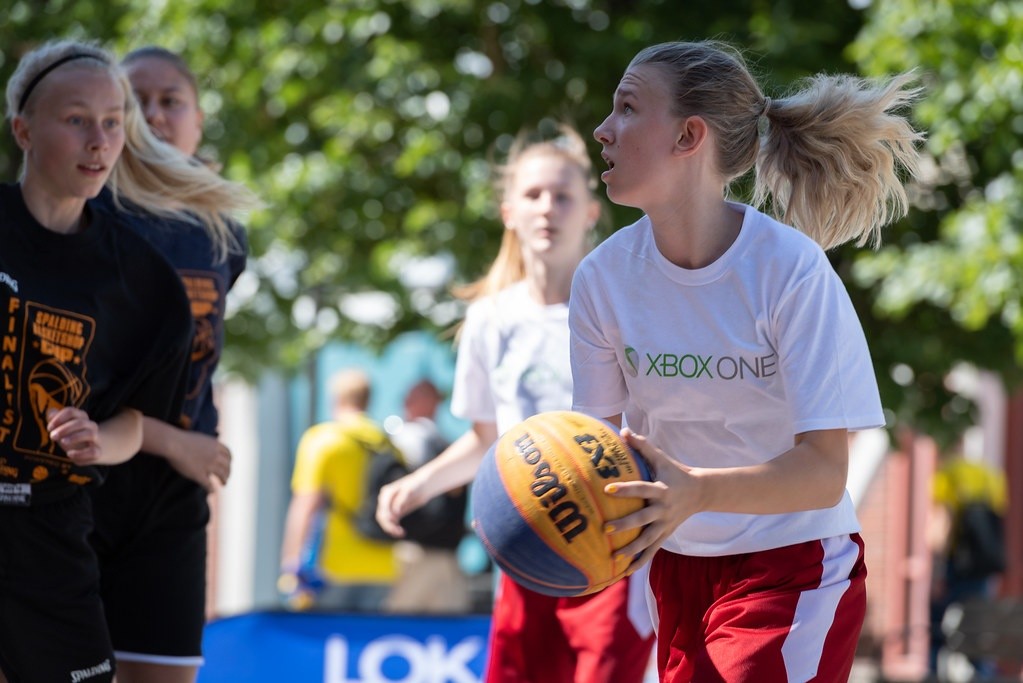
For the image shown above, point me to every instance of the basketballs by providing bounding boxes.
[471,409,651,598]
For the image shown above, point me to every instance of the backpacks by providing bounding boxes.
[328,431,414,541]
[415,436,467,549]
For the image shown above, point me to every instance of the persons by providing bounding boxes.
[0,40,251,683]
[272,369,474,612]
[375,121,657,683]
[568,38,927,683]
